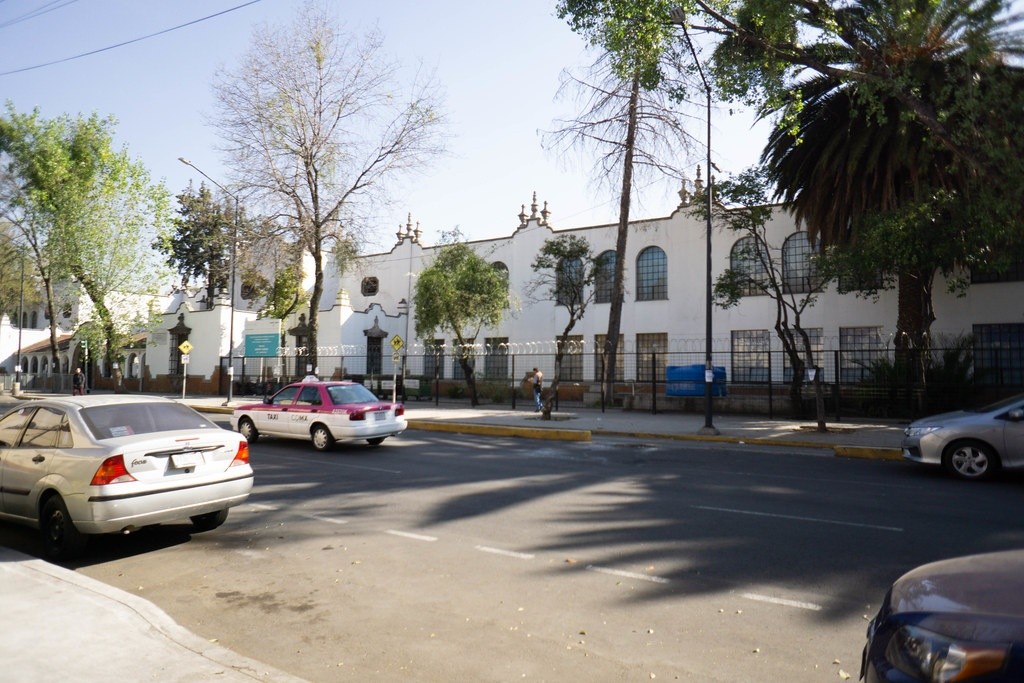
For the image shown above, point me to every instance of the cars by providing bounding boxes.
[900,394,1024,482]
[231,375,408,452]
[858,548,1024,683]
[0,394,254,552]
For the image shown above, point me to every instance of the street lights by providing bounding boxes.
[669,6,720,437]
[178,157,236,407]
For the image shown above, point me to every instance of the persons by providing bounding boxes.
[72,367,86,397]
[527,367,545,413]
